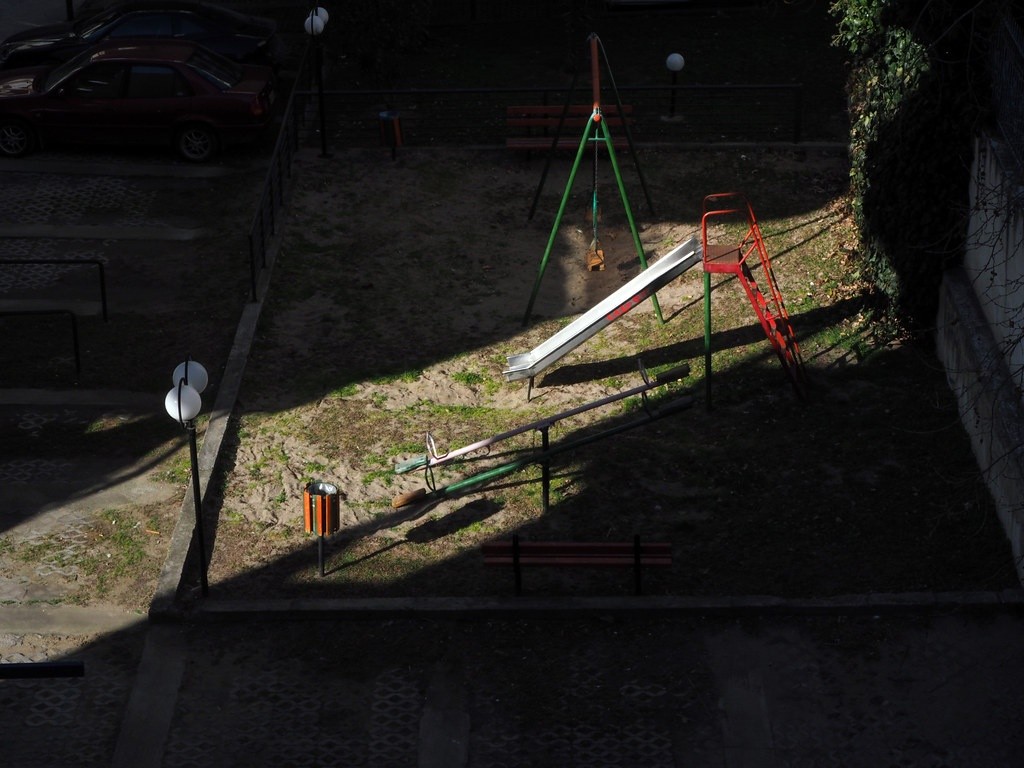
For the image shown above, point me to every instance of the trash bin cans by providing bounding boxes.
[378,112,403,148]
[303,482,340,536]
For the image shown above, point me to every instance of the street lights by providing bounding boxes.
[666,52,685,128]
[165,358,209,598]
[305,1,329,145]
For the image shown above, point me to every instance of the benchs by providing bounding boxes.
[480,534,674,597]
[505,104,633,161]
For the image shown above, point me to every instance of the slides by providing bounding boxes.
[502,238,704,384]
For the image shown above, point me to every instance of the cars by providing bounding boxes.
[0,0,291,77]
[0,32,281,167]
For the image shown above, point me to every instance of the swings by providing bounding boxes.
[586,126,607,272]
